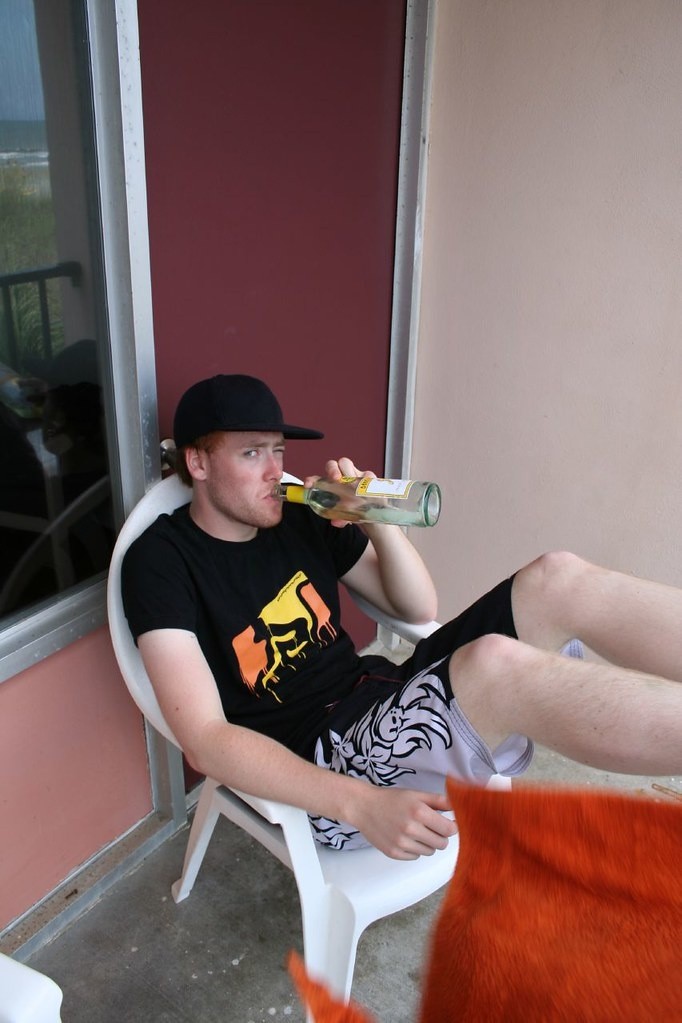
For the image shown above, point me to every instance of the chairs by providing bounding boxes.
[106,471,516,1023]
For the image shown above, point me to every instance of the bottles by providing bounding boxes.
[0,359,52,421]
[272,475,442,526]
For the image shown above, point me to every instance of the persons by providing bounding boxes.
[118,372,682,864]
[0,378,116,604]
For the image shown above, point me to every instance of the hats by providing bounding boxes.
[21,338,107,410]
[173,374,325,447]
